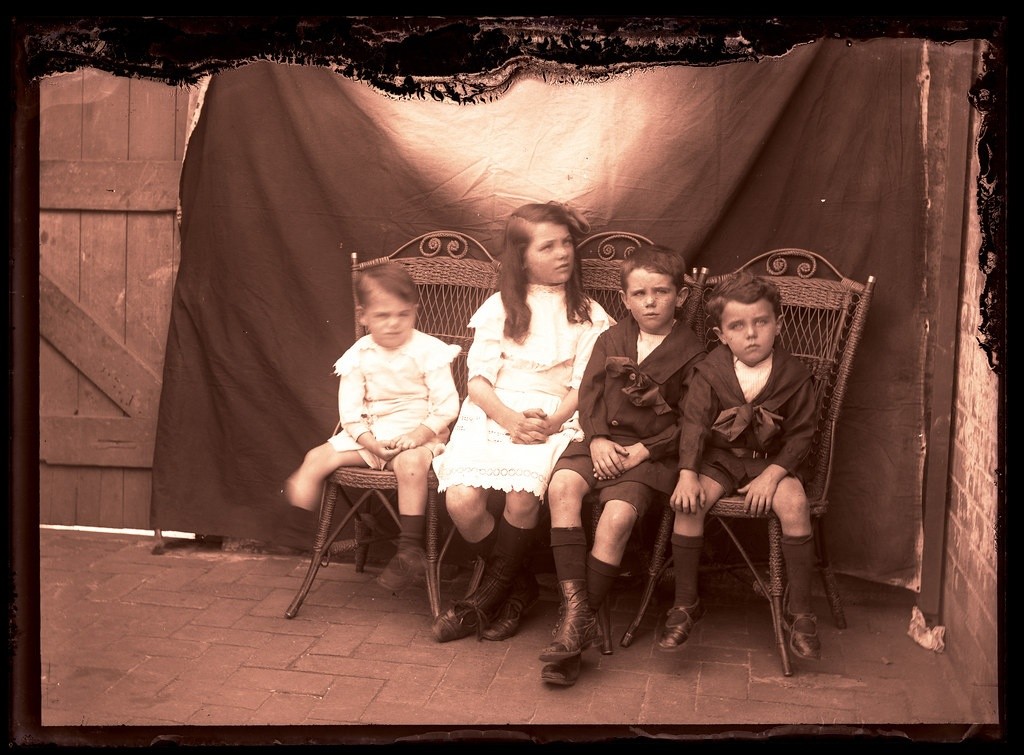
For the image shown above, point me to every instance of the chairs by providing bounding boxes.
[285,231,874,677]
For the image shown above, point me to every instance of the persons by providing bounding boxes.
[537,245,709,687]
[430,203,618,643]
[270,263,461,592]
[656,276,821,658]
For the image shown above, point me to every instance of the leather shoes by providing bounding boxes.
[431,576,541,643]
[654,594,709,654]
[781,600,822,662]
[378,542,426,592]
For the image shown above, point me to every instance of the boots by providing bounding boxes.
[538,578,605,687]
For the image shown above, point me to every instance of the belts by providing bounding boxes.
[719,446,778,460]
